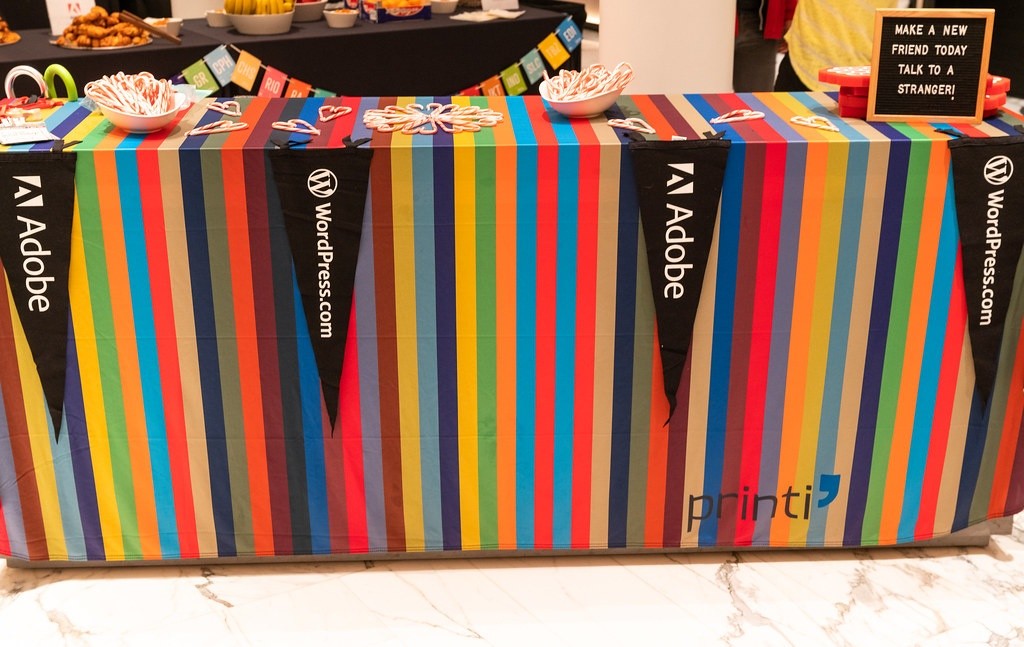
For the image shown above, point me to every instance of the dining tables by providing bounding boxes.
[0,91,1024,570]
[0,5,586,97]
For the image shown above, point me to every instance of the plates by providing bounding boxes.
[0,35,22,46]
[51,34,153,50]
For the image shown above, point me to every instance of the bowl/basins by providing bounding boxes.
[539,76,625,120]
[145,15,184,38]
[223,8,296,34]
[206,10,234,27]
[291,0,329,22]
[94,91,186,134]
[323,9,360,29]
[431,0,459,14]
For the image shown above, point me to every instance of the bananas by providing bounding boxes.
[225,0,295,16]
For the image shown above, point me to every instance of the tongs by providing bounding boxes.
[119,10,182,45]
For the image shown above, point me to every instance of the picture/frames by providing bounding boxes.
[867,9,996,123]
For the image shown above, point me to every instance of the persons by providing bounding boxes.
[772,0,910,92]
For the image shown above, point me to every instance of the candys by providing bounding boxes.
[828,65,873,76]
[271,118,321,135]
[205,101,242,117]
[318,105,352,123]
[710,108,766,125]
[541,63,637,105]
[791,114,839,133]
[606,118,657,135]
[184,120,249,137]
[363,103,504,135]
[85,72,176,116]
